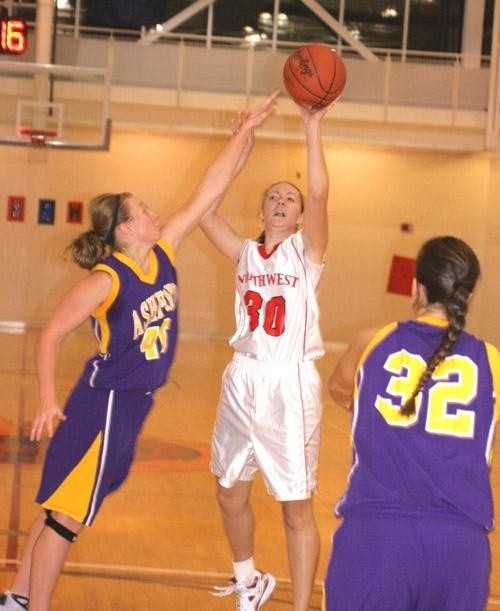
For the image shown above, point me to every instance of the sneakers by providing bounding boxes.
[209,568,277,611]
[0,588,31,611]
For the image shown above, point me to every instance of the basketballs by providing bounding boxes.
[284,45,346,110]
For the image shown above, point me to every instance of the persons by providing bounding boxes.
[191,94,342,611]
[0,89,283,611]
[319,233,500,611]
